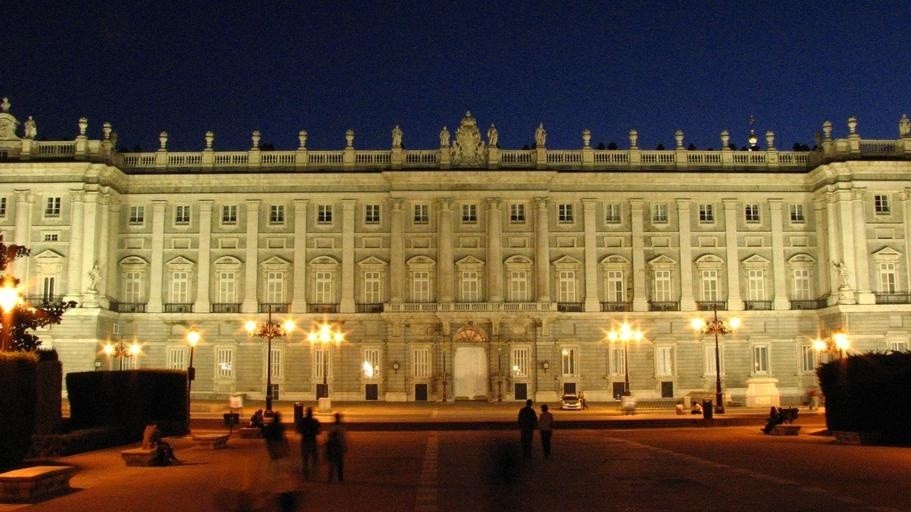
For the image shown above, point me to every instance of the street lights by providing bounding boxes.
[246,306,294,418]
[606,322,645,395]
[691,304,742,414]
[101,333,142,369]
[185,330,202,402]
[0,277,19,348]
[810,331,847,362]
[308,327,343,402]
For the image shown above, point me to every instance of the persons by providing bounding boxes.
[516,398,537,462]
[675,402,686,414]
[389,123,403,150]
[139,417,185,464]
[321,411,347,482]
[1,97,12,115]
[247,408,265,429]
[759,407,786,435]
[487,123,500,147]
[23,114,38,141]
[691,401,705,414]
[295,405,321,480]
[537,405,553,461]
[258,410,291,461]
[534,121,549,145]
[439,126,452,148]
[460,109,477,126]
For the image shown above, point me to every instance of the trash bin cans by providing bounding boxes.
[702,399,712,419]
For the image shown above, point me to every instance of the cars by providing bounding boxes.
[560,395,582,410]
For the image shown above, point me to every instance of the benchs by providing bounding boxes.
[191,433,230,450]
[121,448,159,467]
[775,425,801,435]
[241,427,261,438]
[830,430,874,445]
[0,466,78,501]
